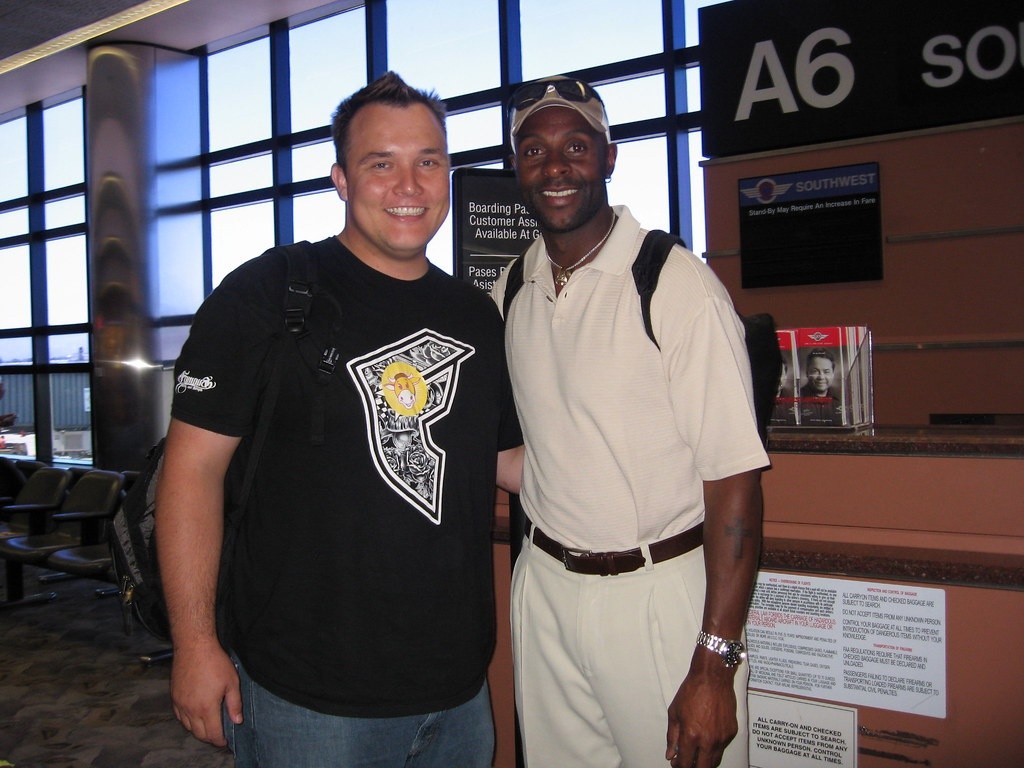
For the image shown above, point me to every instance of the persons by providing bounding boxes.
[801,348,841,425]
[491,76,770,768]
[769,356,795,425]
[154,72,525,768]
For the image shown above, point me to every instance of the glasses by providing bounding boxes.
[504,77,605,135]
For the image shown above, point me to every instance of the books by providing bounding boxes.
[770,326,875,426]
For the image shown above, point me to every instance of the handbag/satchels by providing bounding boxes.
[108,240,324,643]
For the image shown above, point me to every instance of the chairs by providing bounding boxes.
[0,458,143,637]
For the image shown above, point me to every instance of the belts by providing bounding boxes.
[525,518,705,578]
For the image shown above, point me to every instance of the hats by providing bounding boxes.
[510,75,609,154]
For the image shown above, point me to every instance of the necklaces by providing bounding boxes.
[547,212,616,286]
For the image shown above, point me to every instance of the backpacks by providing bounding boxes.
[498,229,783,461]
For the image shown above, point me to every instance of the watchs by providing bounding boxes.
[697,632,746,668]
